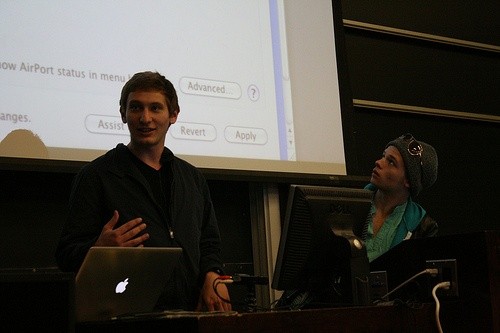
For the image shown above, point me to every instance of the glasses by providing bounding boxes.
[403,132,424,170]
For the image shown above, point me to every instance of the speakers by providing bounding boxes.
[368,232,489,304]
[0,266,74,333]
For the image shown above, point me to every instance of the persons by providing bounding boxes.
[54,72,231,313]
[0,130,51,160]
[352,134,441,270]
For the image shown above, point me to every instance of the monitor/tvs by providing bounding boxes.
[271,184,375,308]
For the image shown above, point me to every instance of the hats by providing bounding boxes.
[386,135,438,192]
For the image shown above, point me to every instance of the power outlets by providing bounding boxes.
[370,271,388,301]
[425,259,459,298]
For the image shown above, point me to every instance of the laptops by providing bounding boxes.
[74,246,182,323]
